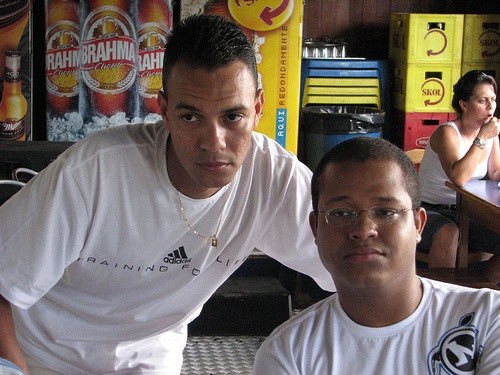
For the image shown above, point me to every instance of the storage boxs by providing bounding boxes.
[389,12,500,173]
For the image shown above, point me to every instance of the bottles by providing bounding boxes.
[0,49,27,141]
[43,0,169,122]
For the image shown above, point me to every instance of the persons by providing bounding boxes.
[414,69,500,268]
[0,15,337,375]
[252,135,500,375]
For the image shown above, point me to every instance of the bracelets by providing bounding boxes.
[472,137,487,149]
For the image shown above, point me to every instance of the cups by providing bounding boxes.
[302,45,345,58]
[204,2,255,46]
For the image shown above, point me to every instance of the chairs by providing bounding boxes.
[405,148,493,263]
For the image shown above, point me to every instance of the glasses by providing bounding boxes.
[317,206,414,226]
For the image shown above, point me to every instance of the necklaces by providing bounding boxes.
[172,170,238,248]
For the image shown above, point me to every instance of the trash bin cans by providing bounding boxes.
[303,102,386,180]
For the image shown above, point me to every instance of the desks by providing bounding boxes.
[445,180,500,268]
